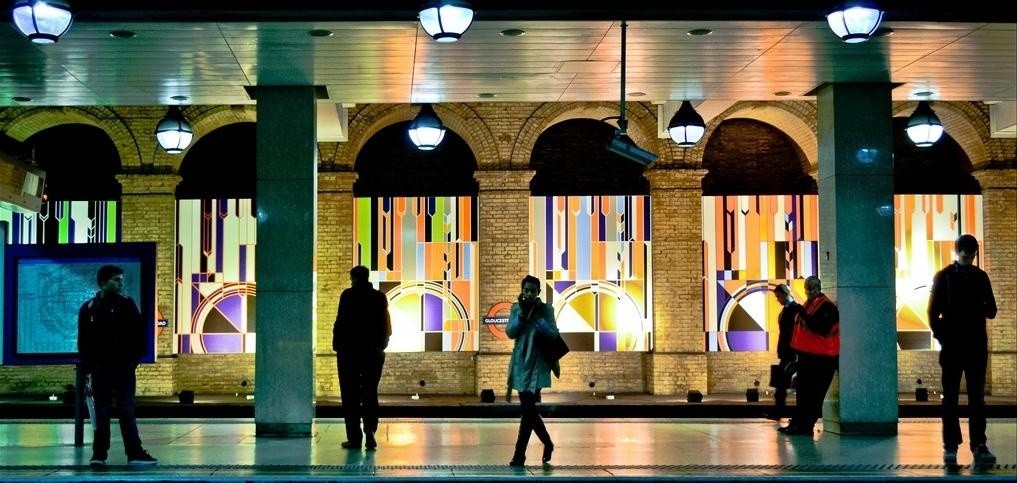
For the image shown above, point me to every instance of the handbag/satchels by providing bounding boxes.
[535,330,570,366]
[769,364,798,389]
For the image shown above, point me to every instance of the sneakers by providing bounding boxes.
[126,450,159,466]
[973,445,997,462]
[943,446,958,465]
[91,449,109,466]
[764,412,815,437]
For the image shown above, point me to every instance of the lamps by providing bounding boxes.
[9,0,75,47]
[825,1,886,45]
[414,2,478,46]
[153,102,193,154]
[904,90,944,148]
[406,102,447,152]
[666,94,707,150]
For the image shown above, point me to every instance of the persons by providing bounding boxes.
[775,274,840,437]
[760,281,806,423]
[503,274,570,467]
[925,232,998,472]
[75,263,158,467]
[330,264,391,451]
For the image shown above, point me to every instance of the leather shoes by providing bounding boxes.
[509,450,528,468]
[364,431,378,449]
[542,443,554,464]
[342,441,363,450]
